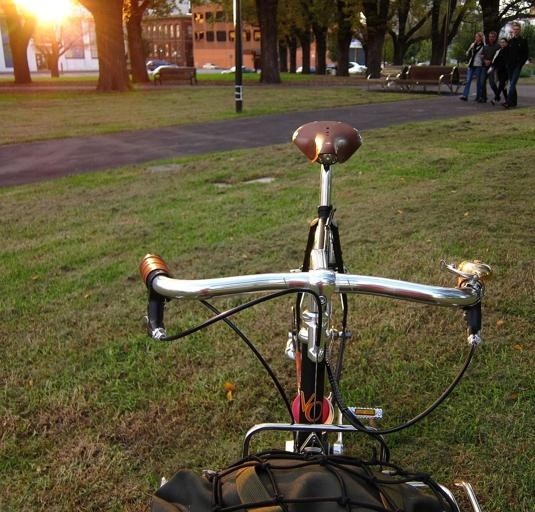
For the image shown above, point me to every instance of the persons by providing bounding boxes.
[487,38,508,106]
[459,32,486,101]
[502,23,529,109]
[476,31,501,103]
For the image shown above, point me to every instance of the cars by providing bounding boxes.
[146,59,175,71]
[221,65,256,74]
[290,60,368,74]
[201,63,217,69]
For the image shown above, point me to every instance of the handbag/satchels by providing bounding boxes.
[149,448,463,512]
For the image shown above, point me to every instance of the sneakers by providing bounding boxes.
[473,97,487,104]
[490,100,495,106]
[501,102,517,109]
[459,94,468,102]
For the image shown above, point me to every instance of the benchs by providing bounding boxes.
[367,64,463,96]
[153,67,198,85]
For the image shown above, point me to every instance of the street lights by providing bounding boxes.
[158,47,163,60]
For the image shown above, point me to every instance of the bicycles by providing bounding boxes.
[132,118,494,512]
